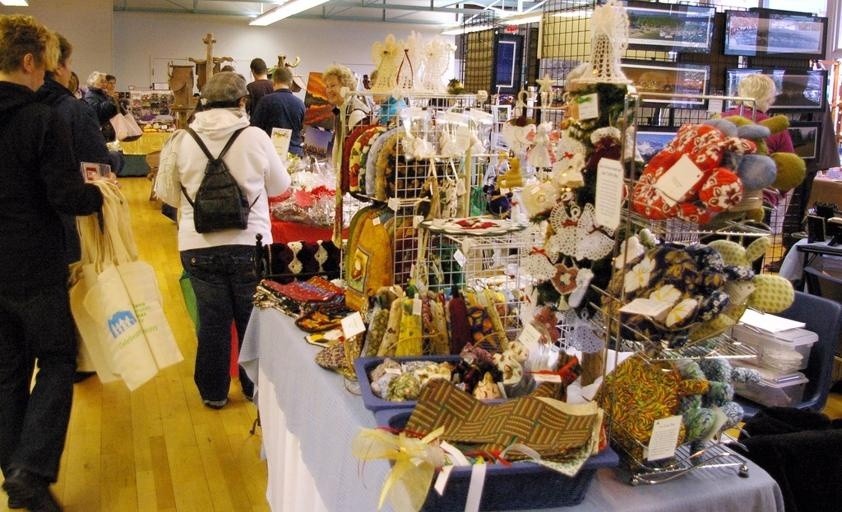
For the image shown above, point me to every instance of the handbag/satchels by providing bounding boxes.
[110,113,143,142]
[69,180,184,392]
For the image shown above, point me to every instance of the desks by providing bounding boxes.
[113,125,174,177]
[260,201,349,283]
[237,303,786,512]
[796,243,842,302]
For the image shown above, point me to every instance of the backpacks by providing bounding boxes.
[179,126,263,233]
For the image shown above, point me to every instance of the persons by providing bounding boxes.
[322,64,370,143]
[222,58,305,157]
[0,15,124,512]
[773,70,819,105]
[154,71,292,410]
[70,70,123,152]
[44,32,124,382]
[700,74,794,275]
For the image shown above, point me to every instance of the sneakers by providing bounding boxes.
[204,393,227,408]
[239,366,254,401]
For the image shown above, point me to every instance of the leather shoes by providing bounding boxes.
[2,467,64,512]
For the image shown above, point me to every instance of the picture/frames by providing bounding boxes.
[494,33,525,96]
[619,58,711,110]
[616,1,716,55]
[723,9,828,60]
[627,125,681,177]
[722,67,828,113]
[787,121,823,163]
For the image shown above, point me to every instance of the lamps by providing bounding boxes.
[248,0,330,27]
[441,1,543,35]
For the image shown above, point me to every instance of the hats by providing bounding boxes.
[201,71,250,103]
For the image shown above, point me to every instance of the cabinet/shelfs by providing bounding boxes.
[128,89,175,124]
[339,87,774,486]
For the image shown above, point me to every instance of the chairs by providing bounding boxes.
[732,289,842,423]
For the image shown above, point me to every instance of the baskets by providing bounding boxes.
[352,356,619,512]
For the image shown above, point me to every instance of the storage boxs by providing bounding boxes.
[730,360,810,408]
[731,323,819,374]
